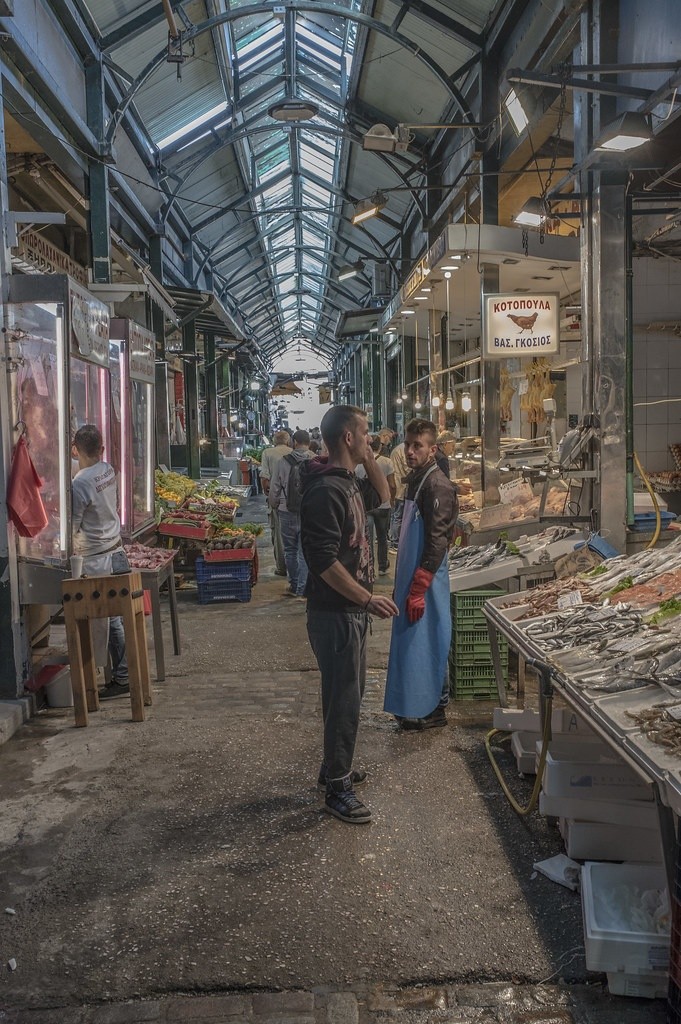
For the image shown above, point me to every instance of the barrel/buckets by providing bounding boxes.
[45,665,74,707]
[48,624,67,647]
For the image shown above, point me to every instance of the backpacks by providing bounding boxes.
[283,454,313,513]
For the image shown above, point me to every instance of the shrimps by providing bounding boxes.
[624,700,681,759]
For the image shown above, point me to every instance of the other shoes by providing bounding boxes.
[274,570,287,576]
[287,586,297,597]
[297,596,308,602]
[398,706,447,731]
[388,547,398,554]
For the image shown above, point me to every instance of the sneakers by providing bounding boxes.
[378,559,390,576]
[324,770,371,823]
[98,680,131,701]
[317,765,368,792]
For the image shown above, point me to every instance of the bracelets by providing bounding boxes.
[365,595,373,610]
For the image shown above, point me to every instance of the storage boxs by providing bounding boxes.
[158,484,261,604]
[445,566,681,1000]
[624,490,677,533]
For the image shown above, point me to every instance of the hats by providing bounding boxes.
[436,430,454,444]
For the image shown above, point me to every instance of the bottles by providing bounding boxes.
[454,422,461,438]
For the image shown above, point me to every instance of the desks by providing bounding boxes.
[127,546,180,681]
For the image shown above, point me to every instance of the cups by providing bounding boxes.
[69,555,83,578]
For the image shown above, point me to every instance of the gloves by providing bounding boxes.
[404,567,432,625]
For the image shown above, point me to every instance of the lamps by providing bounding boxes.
[363,122,488,155]
[337,257,420,282]
[353,184,461,223]
[513,197,554,228]
[503,83,538,139]
[369,322,379,332]
[592,109,656,154]
[267,10,319,121]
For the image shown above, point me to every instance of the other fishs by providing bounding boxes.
[499,535,681,698]
[449,538,506,572]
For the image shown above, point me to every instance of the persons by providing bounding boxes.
[354,434,397,576]
[380,426,413,554]
[299,405,401,823]
[382,417,453,730]
[259,430,294,576]
[435,431,456,480]
[259,426,329,456]
[269,430,318,601]
[71,424,131,701]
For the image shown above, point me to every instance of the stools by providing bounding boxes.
[62,570,151,727]
